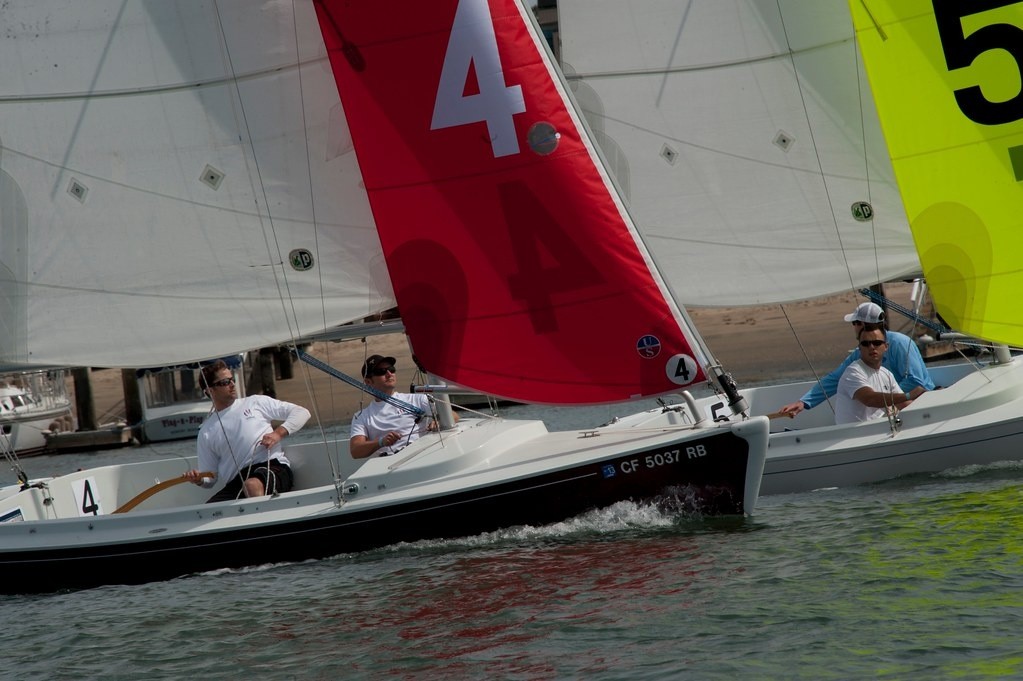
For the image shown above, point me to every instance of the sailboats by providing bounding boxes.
[409,0,1023,494]
[0,0,774,591]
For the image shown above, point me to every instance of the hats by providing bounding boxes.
[844,302,885,324]
[361,354,396,377]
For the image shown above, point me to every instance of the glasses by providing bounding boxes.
[208,376,236,388]
[858,340,886,347]
[372,366,395,377]
[852,321,857,326]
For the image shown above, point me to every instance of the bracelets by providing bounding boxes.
[906,392,910,400]
[378,436,384,447]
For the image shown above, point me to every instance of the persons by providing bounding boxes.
[182,359,312,504]
[778,302,935,425]
[350,354,459,459]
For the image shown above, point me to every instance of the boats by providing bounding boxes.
[1,368,77,460]
[134,351,247,445]
